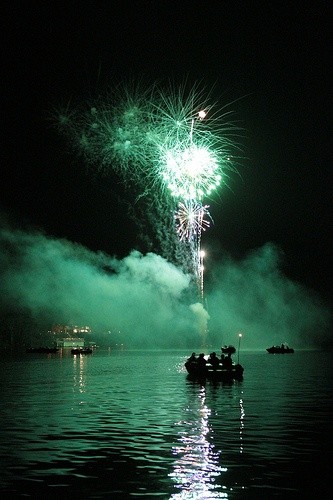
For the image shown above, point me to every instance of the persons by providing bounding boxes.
[188,352,232,364]
[281,344,290,349]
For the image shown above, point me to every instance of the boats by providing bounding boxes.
[43,347,59,354]
[266,345,294,354]
[185,358,244,380]
[70,345,93,355]
